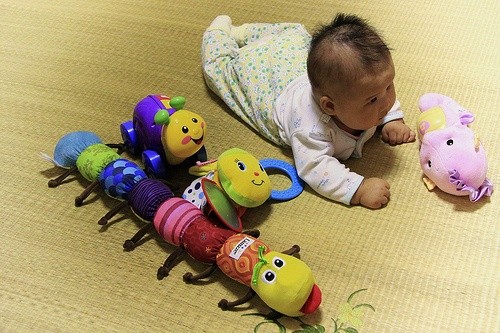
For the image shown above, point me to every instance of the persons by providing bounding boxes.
[201,13,416,210]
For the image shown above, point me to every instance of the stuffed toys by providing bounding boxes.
[416,93,493,203]
[40,130,321,317]
